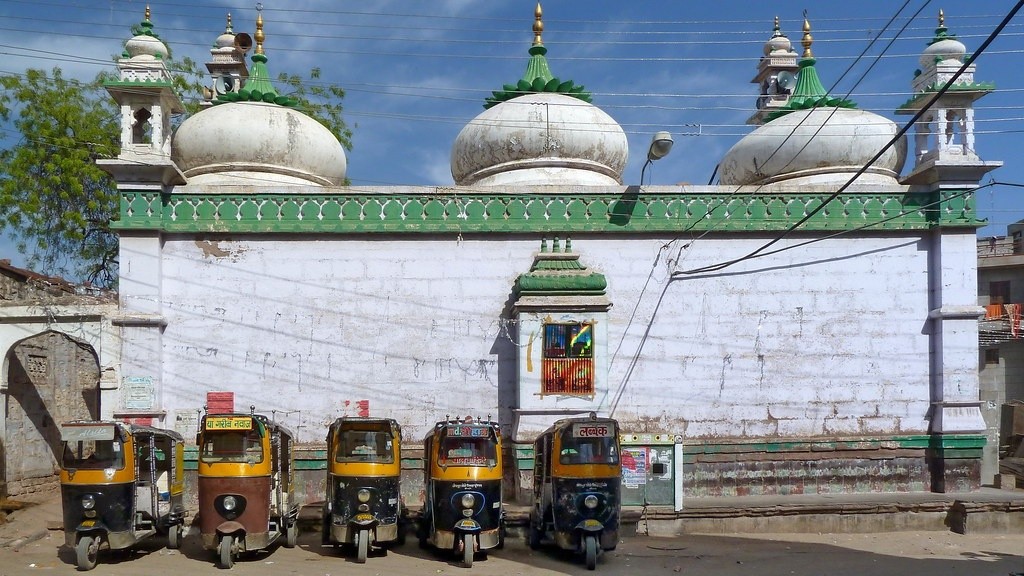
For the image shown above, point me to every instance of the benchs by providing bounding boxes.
[140,469,171,504]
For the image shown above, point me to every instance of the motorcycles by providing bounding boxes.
[418,413,507,569]
[529,411,624,570]
[320,415,405,564]
[54,419,190,571]
[198,404,303,569]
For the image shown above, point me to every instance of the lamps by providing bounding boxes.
[641,131,673,185]
